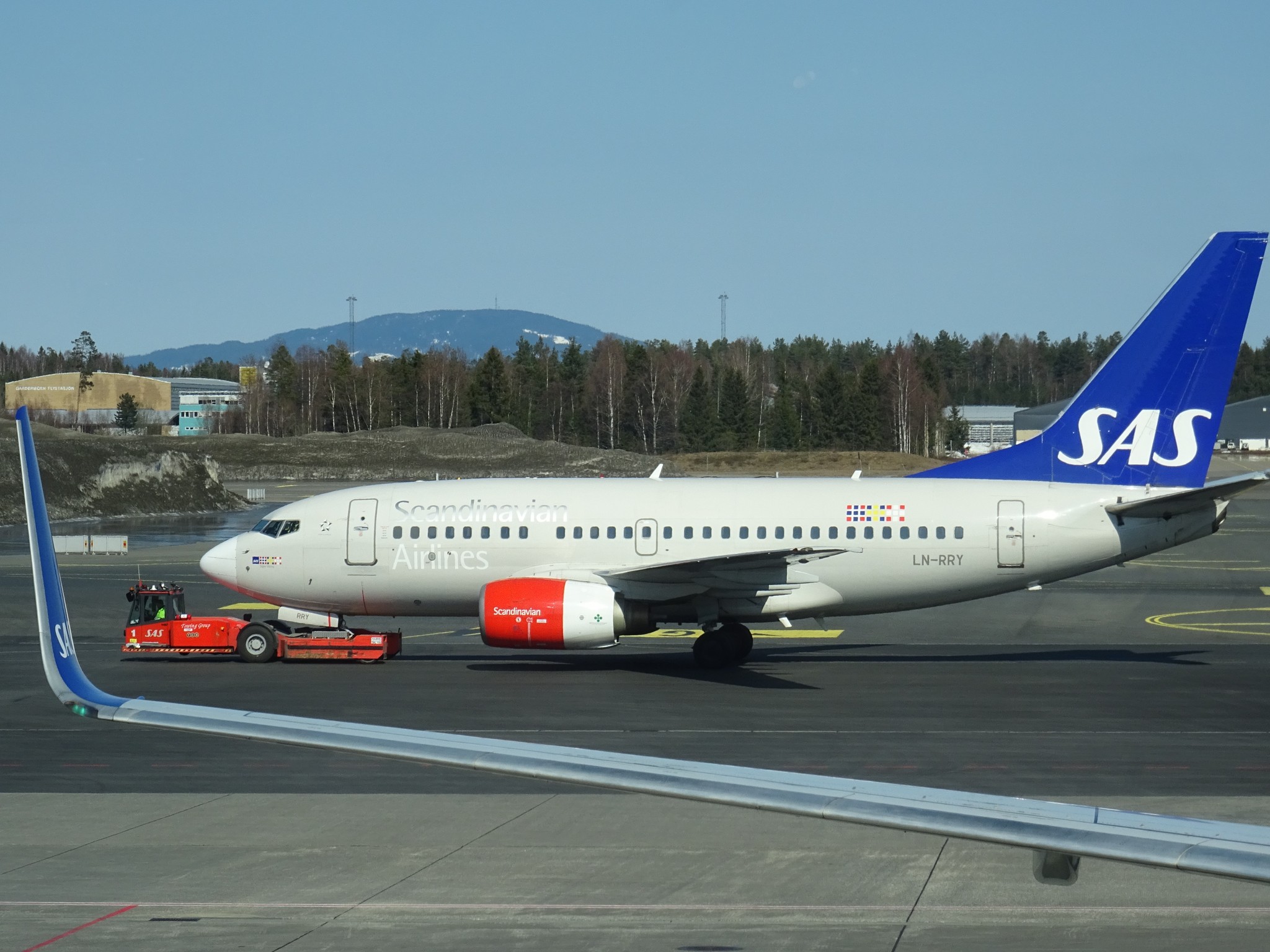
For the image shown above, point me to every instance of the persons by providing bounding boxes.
[153,600,165,621]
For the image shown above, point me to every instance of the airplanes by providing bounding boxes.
[198,226,1270,677]
